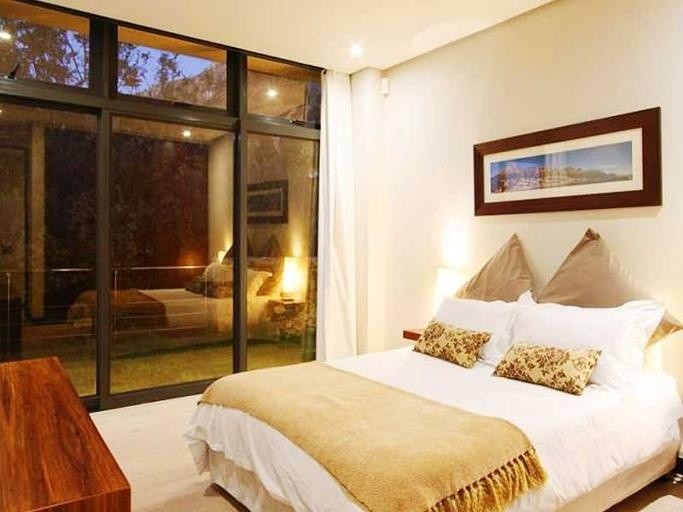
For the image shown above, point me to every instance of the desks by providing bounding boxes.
[0,356,131,512]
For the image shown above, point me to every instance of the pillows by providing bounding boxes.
[412,228,683,395]
[186,235,284,299]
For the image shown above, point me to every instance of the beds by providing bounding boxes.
[68,287,281,352]
[185,343,682,512]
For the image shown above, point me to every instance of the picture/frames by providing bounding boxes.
[247,180,289,224]
[473,107,663,217]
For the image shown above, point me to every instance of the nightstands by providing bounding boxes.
[403,329,420,341]
[269,299,306,338]
